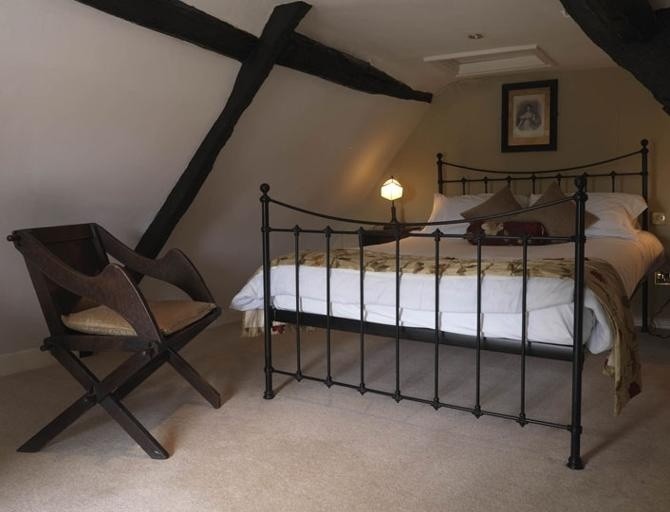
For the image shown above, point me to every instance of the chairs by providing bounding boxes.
[6,222,222,464]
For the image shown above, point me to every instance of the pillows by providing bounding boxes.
[417,179,649,247]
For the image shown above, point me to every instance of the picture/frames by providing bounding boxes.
[501,78,558,154]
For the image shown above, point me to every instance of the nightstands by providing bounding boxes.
[358,225,421,246]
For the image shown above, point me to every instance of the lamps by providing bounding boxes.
[380,174,404,231]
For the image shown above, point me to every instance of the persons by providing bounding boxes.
[518,105,536,130]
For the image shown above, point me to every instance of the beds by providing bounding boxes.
[262,138,651,466]
